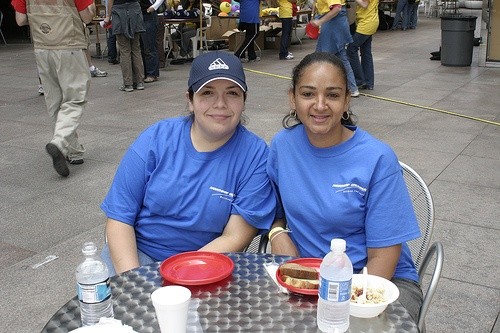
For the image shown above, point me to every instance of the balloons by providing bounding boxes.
[218,0,240,16]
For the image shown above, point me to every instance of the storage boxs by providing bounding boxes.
[264,28,282,50]
[222,30,264,51]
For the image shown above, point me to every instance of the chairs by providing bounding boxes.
[258,161,444,333]
[190,16,211,58]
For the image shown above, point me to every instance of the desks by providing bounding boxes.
[218,11,312,58]
[82,18,109,59]
[164,19,207,68]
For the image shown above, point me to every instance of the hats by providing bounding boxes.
[188,50,248,94]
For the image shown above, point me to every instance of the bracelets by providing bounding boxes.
[268,226,292,243]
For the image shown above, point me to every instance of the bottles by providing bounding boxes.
[75,242,114,327]
[317,238,353,333]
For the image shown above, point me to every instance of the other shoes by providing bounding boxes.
[351,90,360,96]
[249,57,260,62]
[66,155,84,164]
[144,77,157,82]
[108,59,118,64]
[358,82,369,89]
[288,51,293,55]
[45,143,70,176]
[241,58,249,63]
[280,55,294,60]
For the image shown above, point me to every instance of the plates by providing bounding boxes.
[68,327,136,333]
[276,258,323,295]
[160,251,233,285]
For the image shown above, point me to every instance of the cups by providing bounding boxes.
[151,285,192,333]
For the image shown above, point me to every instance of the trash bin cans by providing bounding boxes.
[440,15,478,67]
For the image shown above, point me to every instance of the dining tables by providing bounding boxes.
[39,252,420,333]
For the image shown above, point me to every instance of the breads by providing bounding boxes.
[280,263,320,289]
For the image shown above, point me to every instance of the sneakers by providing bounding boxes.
[38,84,44,94]
[134,82,144,90]
[90,67,107,77]
[120,85,134,92]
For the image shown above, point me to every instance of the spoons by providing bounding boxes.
[358,267,367,303]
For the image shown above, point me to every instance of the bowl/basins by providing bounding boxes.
[349,274,400,318]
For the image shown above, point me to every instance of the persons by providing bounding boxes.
[100,52,277,278]
[11,0,93,177]
[390,0,420,32]
[38,3,108,96]
[266,52,424,325]
[138,0,165,83]
[279,0,297,59]
[166,0,207,65]
[311,0,360,97]
[234,0,260,63]
[104,0,146,91]
[346,0,379,90]
